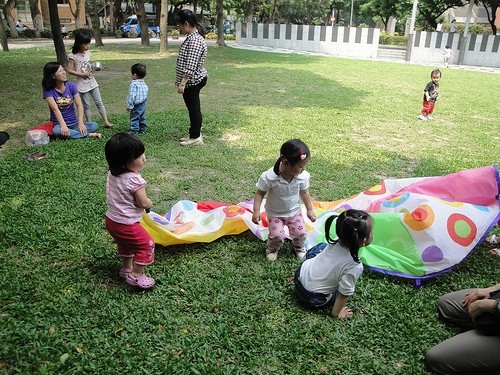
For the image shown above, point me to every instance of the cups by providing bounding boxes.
[94,61,101,70]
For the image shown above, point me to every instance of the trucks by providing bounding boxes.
[119,15,160,38]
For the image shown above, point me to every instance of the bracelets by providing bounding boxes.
[180,83,185,86]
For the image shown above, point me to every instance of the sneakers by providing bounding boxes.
[180,136,203,146]
[179,133,202,142]
[296,250,306,260]
[266,248,277,261]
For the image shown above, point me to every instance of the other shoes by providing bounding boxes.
[420,115,425,121]
[26,153,48,162]
[427,115,432,120]
[24,150,44,160]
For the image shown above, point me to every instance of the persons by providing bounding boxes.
[294,209,375,319]
[105,133,156,289]
[441,44,456,68]
[16,19,24,32]
[425,283,500,375]
[42,62,102,140]
[61,24,68,39]
[251,139,316,262]
[65,30,114,128]
[417,69,442,120]
[484,234,500,257]
[126,63,149,136]
[175,9,208,146]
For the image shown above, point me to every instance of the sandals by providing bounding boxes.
[125,270,155,287]
[119,267,131,278]
